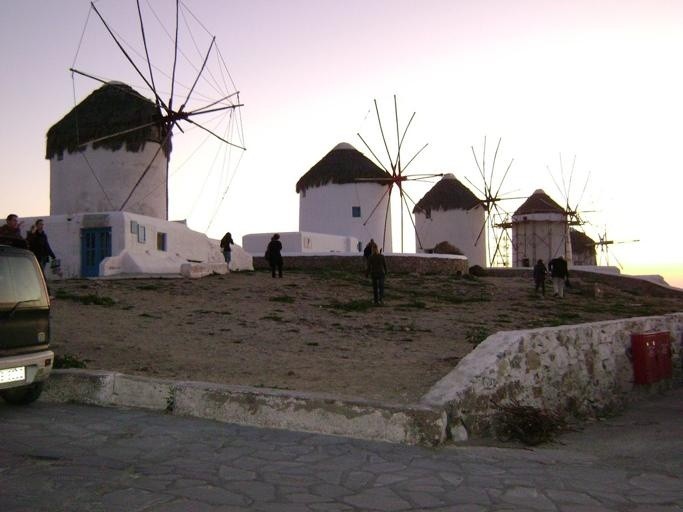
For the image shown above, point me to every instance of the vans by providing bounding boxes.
[0,242,54,403]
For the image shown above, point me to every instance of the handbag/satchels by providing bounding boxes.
[220,248,225,252]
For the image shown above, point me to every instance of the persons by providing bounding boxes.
[0,214,57,300]
[220,232,234,272]
[365,249,387,306]
[266,233,283,278]
[532,259,547,296]
[363,239,378,278]
[548,255,568,298]
